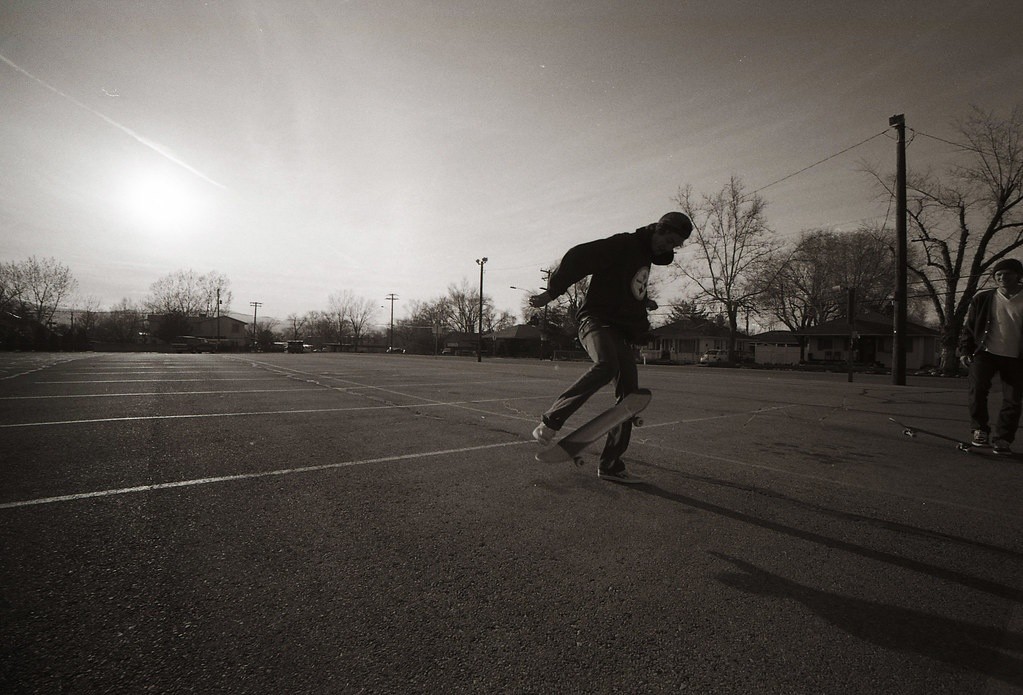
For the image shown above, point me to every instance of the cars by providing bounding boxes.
[312,347,327,352]
[701,348,727,367]
[441,348,473,355]
[386,347,406,354]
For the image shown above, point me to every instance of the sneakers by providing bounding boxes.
[971,430,990,446]
[597,466,646,483]
[992,439,1012,455]
[532,422,556,446]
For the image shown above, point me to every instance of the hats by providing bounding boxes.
[992,259,1023,281]
[657,212,693,239]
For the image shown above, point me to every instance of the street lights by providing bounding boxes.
[832,284,853,382]
[510,286,550,361]
[476,257,487,363]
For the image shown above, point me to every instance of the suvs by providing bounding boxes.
[172,336,218,353]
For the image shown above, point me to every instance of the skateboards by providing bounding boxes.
[888,416,995,456]
[533,386,654,469]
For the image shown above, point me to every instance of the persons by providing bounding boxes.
[530,211,693,483]
[957,259,1023,455]
